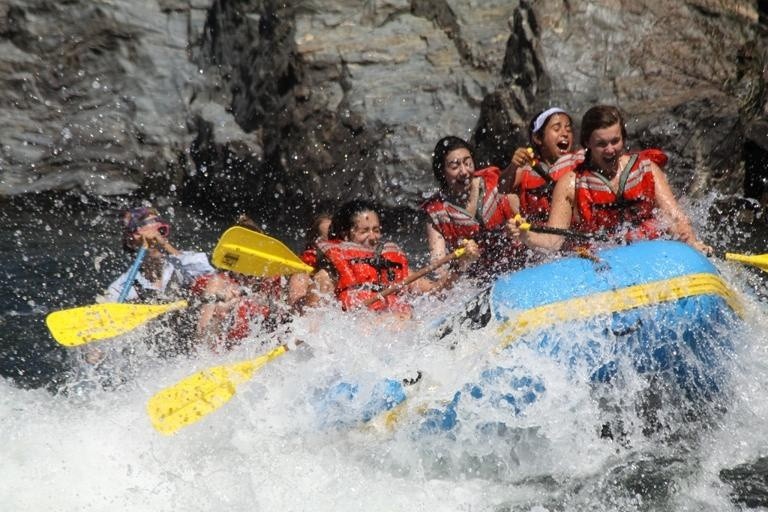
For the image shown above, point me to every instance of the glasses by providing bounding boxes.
[133,225,169,242]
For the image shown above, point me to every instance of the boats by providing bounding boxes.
[67,239,752,473]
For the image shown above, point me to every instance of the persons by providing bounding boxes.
[503,109,671,267]
[282,214,337,317]
[79,206,214,367]
[192,217,306,357]
[505,104,712,265]
[307,200,457,327]
[420,135,522,323]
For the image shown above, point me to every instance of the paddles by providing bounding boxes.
[148,248,464,436]
[45,281,285,347]
[515,216,768,271]
[211,226,315,277]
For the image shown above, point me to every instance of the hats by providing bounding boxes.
[122,207,163,251]
